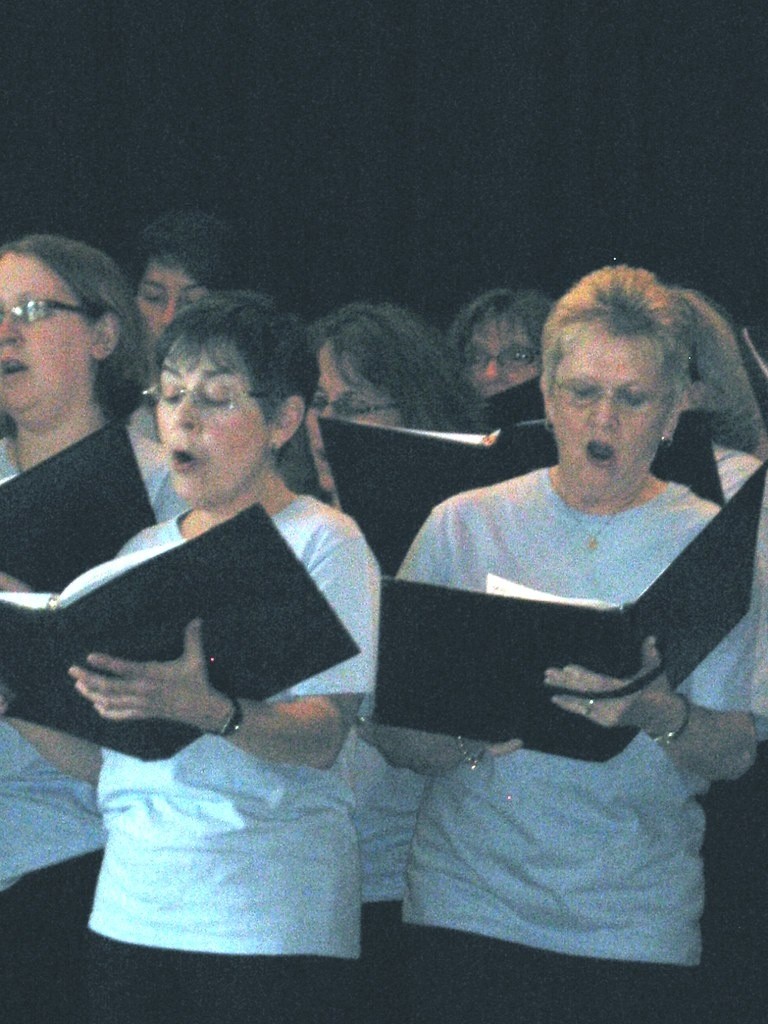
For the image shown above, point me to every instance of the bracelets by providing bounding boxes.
[456,735,486,768]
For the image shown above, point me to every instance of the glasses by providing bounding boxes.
[458,345,542,376]
[0,300,100,325]
[311,389,404,421]
[141,379,273,412]
[550,370,669,413]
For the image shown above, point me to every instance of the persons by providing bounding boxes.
[355,269,767,1024]
[0,234,480,1024]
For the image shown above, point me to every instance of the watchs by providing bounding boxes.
[645,693,691,746]
[200,695,242,737]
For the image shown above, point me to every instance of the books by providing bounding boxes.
[1,502,360,761]
[375,461,768,762]
[0,420,156,596]
[318,413,724,576]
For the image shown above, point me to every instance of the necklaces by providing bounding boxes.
[553,477,619,550]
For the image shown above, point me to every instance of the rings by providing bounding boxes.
[587,699,596,709]
